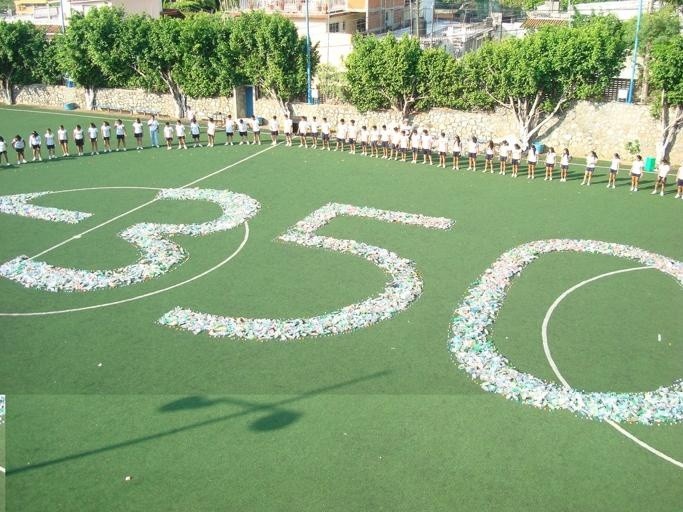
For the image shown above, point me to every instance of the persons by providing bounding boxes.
[0,105,683,200]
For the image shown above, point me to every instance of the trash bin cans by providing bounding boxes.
[65,103,74,110]
[645,157,656,172]
[257,117,263,125]
[533,142,545,154]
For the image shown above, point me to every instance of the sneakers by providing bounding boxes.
[651,191,683,200]
[360,152,409,161]
[411,159,478,171]
[606,183,639,192]
[47,154,70,160]
[6,158,43,167]
[482,168,518,178]
[298,143,331,151]
[271,140,294,147]
[178,141,262,148]
[527,174,592,185]
[150,144,174,150]
[90,147,146,156]
[335,147,357,155]
